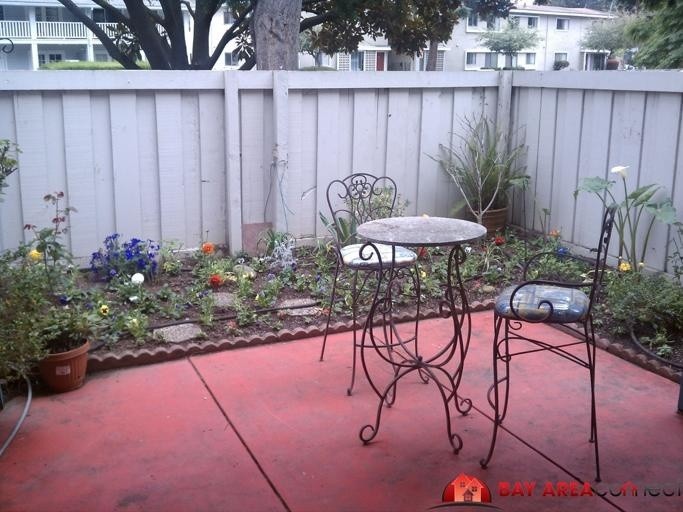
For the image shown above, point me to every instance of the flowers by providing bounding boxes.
[27,243,109,319]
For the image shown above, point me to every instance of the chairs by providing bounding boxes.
[320,172,432,385]
[479,201,618,483]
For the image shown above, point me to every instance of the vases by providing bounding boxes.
[33,335,88,391]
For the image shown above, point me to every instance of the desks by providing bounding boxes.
[356,215,488,455]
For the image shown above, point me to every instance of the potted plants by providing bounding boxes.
[433,113,533,240]
